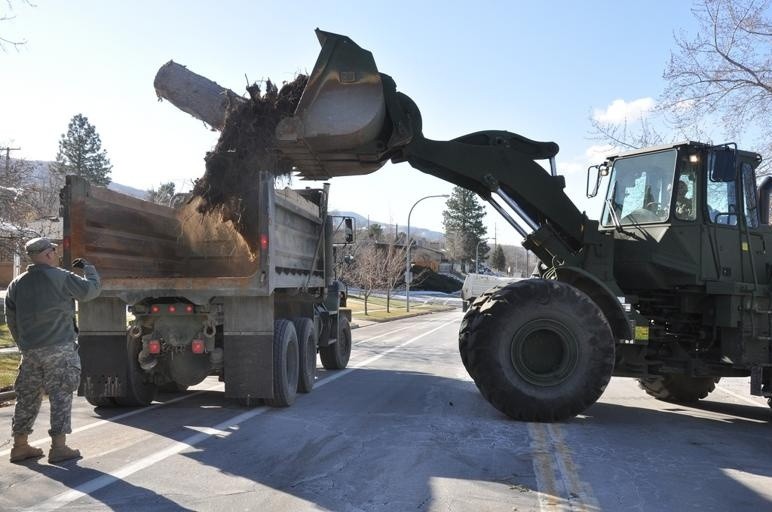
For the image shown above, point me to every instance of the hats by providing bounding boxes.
[25,238,57,256]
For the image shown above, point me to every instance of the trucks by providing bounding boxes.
[55,174,365,413]
[459,260,550,315]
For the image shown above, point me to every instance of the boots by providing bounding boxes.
[10,435,43,461]
[48,433,80,462]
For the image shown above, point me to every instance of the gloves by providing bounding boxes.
[73,258,89,268]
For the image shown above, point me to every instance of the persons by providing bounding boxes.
[4,236,101,464]
[664,180,693,217]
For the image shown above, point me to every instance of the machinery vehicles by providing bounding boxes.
[274,19,772,420]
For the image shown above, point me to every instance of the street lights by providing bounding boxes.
[406,192,451,313]
[474,237,498,271]
